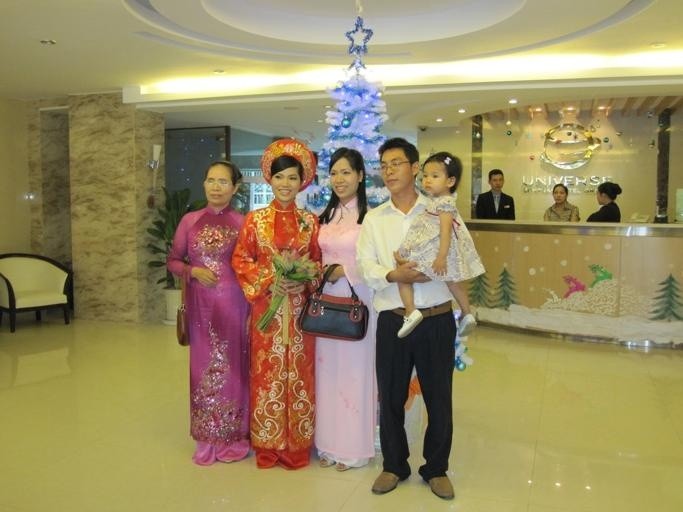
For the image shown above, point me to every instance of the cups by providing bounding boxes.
[203,178,235,186]
[380,160,412,169]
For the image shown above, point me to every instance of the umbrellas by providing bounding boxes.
[373,473,400,494]
[430,476,455,500]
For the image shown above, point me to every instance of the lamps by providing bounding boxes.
[0,253,72,333]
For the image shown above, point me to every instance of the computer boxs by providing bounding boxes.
[418,125,429,132]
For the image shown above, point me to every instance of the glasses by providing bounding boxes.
[596,104,611,112]
[508,98,519,105]
[458,108,466,115]
[567,106,575,112]
[436,117,443,123]
[533,106,544,114]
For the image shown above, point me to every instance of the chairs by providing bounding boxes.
[458,314,477,337]
[397,309,424,338]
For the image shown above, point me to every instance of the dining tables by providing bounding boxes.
[145,185,206,325]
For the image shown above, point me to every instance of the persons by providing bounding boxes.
[231,139,323,472]
[544,184,580,222]
[476,168,515,220]
[312,147,376,473]
[587,183,622,222]
[167,160,251,466]
[356,138,457,500]
[393,151,485,339]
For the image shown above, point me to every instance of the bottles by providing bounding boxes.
[262,138,317,192]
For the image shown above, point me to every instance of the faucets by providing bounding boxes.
[337,462,349,469]
[319,457,335,468]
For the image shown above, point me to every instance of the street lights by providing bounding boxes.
[177,264,189,347]
[299,264,369,341]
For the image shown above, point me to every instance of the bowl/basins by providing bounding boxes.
[269,205,296,213]
